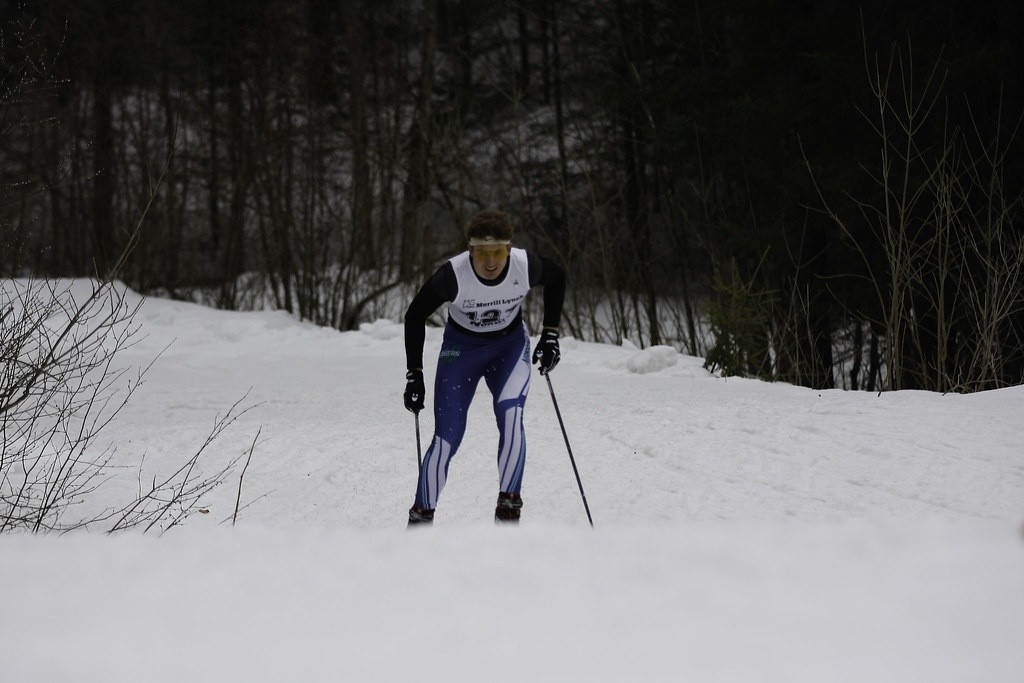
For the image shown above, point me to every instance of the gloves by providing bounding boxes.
[403,369,425,413]
[532,328,561,376]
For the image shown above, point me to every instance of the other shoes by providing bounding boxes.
[406,503,436,531]
[494,491,523,522]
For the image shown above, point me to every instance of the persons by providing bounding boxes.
[405,209,568,527]
